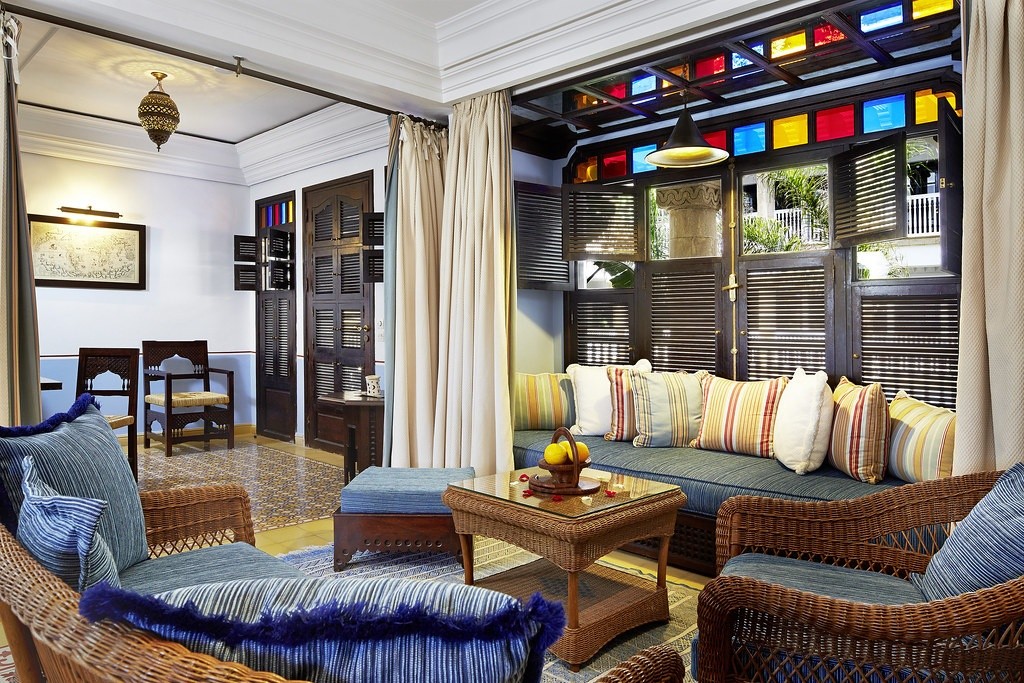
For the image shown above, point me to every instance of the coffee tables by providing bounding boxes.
[441,465,686,673]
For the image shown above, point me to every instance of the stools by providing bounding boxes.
[332,465,474,570]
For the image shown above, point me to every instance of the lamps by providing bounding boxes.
[644,60,730,165]
[138,73,181,151]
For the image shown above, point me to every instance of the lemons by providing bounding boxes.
[544,440,590,464]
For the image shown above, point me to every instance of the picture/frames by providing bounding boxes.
[26,213,146,289]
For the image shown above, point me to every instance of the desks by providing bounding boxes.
[319,390,384,484]
[40,378,62,390]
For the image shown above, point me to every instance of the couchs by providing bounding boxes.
[695,469,1024,683]
[511,429,949,576]
[0,484,686,682]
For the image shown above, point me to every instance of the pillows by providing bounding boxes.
[773,367,834,474]
[628,368,711,448]
[0,393,149,574]
[910,461,1024,609]
[605,366,647,442]
[17,455,120,594]
[512,372,576,430]
[564,359,653,436]
[80,574,565,682]
[887,390,956,481]
[832,375,891,484]
[688,372,788,460]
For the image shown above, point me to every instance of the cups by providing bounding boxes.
[365,374,382,401]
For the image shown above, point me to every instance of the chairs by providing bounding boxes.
[74,347,138,486]
[142,340,234,456]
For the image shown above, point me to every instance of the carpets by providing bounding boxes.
[99,441,361,551]
[276,535,702,681]
